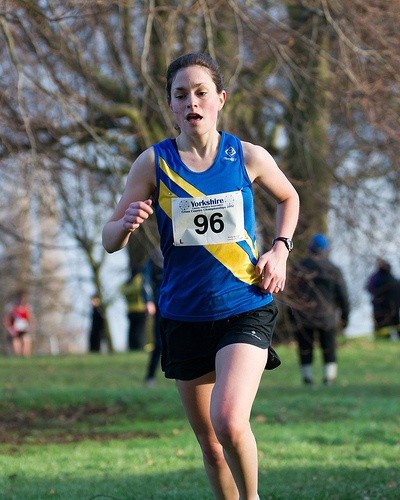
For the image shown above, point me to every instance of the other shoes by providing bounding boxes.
[323,378,335,386]
[302,376,314,386]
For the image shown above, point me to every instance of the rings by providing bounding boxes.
[276,284,281,289]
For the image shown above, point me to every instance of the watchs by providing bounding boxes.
[272,237,293,253]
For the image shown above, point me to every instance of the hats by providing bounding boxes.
[307,233,330,254]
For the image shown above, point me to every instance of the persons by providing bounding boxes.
[3,290,33,358]
[287,234,351,387]
[89,294,106,353]
[122,268,150,351]
[101,52,300,500]
[368,257,400,339]
